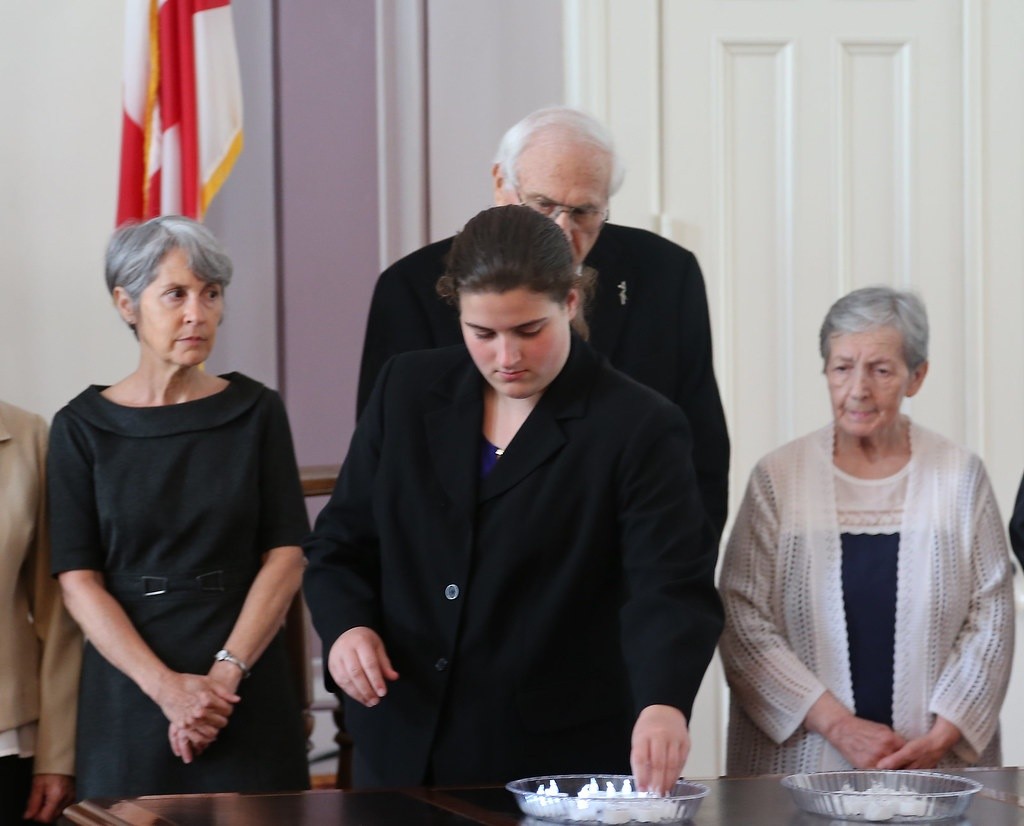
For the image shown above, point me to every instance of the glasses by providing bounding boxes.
[500,162,610,234]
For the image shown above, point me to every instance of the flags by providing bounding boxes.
[115,0,246,225]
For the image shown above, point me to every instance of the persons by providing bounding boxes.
[327,107,733,788]
[718,287,1015,774]
[299,204,725,795]
[0,399,84,826]
[41,216,313,797]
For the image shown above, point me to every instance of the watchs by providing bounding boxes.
[213,649,248,676]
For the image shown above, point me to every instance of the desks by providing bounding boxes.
[64,768,1024,826]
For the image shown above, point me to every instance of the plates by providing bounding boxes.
[780,769,984,822]
[506,774,711,825]
